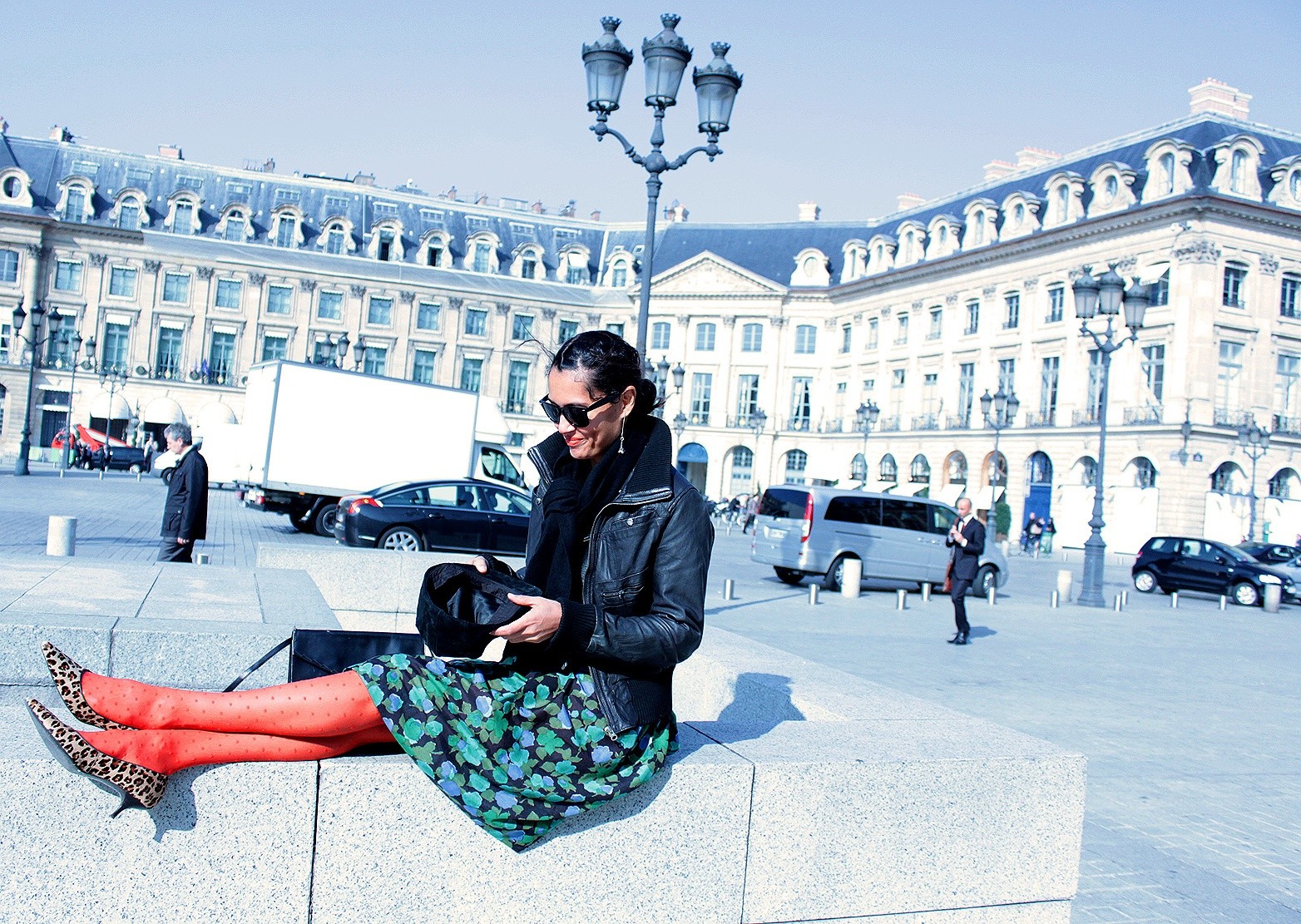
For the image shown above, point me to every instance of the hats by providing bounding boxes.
[415,561,543,659]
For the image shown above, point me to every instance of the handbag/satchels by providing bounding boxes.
[942,560,953,592]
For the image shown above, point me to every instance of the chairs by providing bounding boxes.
[457,492,473,509]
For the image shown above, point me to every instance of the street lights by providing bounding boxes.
[1236,420,1272,542]
[57,327,97,471]
[855,398,881,491]
[1071,261,1154,609]
[978,376,1021,545]
[747,406,767,495]
[11,299,65,476]
[673,411,688,456]
[97,365,128,444]
[580,10,746,382]
[643,354,686,419]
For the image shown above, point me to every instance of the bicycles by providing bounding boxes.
[720,505,748,528]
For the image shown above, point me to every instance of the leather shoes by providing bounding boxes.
[946,629,970,645]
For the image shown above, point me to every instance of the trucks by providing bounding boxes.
[229,357,532,537]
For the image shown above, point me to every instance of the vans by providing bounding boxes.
[152,422,242,487]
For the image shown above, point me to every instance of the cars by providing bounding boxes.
[332,478,533,557]
[1231,540,1301,565]
[1270,554,1301,605]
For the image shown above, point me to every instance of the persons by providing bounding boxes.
[1024,512,1056,556]
[158,423,208,565]
[942,497,985,642]
[24,331,715,853]
[69,440,104,470]
[1293,534,1301,549]
[144,436,158,474]
[1241,534,1246,543]
[708,491,762,534]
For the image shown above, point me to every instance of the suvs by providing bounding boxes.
[75,444,147,475]
[1131,535,1296,607]
[749,480,1010,599]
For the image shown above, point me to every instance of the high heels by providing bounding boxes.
[40,641,142,731]
[24,697,169,819]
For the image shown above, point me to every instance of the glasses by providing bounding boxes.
[539,389,623,428]
